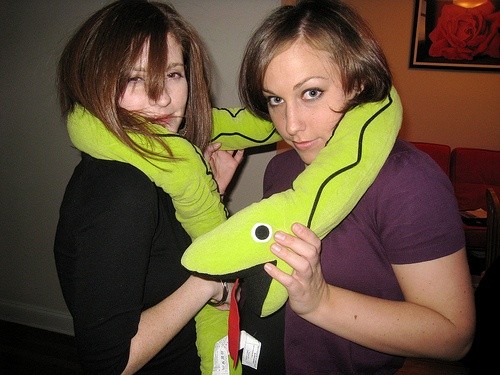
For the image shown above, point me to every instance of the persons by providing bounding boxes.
[53,0,244,375]
[238,0,475,374]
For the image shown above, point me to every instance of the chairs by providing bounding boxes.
[413,143,500,249]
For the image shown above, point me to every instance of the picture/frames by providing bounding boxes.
[409,0,500,71]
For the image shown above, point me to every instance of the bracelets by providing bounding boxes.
[207,277,229,307]
